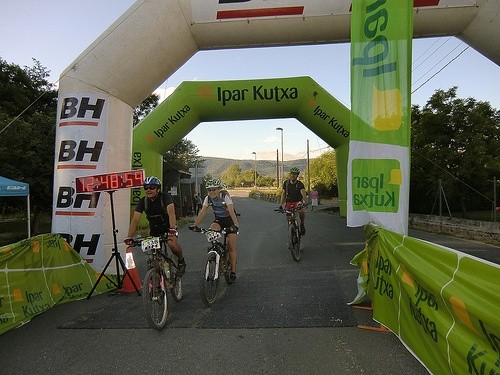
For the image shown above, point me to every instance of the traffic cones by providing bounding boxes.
[120,247,143,293]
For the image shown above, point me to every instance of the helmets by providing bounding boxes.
[205,178,222,189]
[290,167,300,175]
[143,176,161,188]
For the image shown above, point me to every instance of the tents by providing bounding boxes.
[0,176,31,238]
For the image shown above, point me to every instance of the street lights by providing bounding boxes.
[253,152,256,186]
[276,128,284,182]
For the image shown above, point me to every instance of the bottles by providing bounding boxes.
[163,261,171,279]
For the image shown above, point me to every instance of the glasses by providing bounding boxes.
[144,186,155,191]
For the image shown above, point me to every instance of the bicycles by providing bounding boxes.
[127,232,186,330]
[188,225,239,306]
[274,202,309,262]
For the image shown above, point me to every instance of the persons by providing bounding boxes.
[190,178,240,282]
[311,187,319,206]
[123,176,186,301]
[197,193,203,216]
[279,167,308,250]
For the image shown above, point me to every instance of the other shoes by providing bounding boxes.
[176,258,186,275]
[300,223,306,236]
[228,272,236,283]
